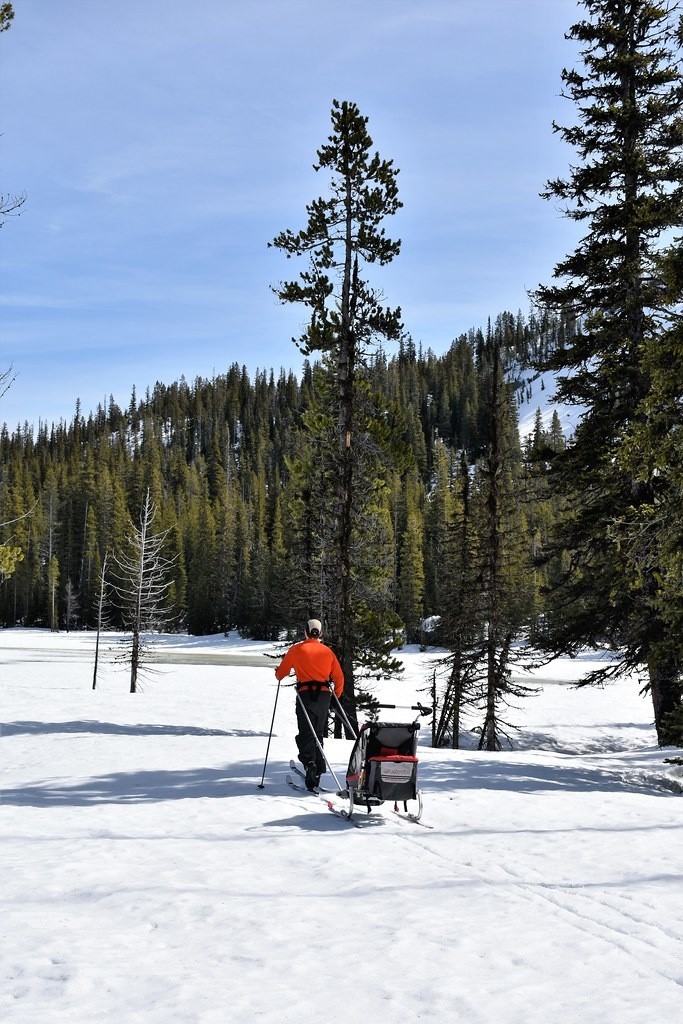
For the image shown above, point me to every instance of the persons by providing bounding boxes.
[275,619,345,790]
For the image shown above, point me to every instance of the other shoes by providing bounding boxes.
[305,761,316,790]
[315,775,320,786]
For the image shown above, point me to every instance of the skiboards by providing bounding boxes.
[283,758,340,806]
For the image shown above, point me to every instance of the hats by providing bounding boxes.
[305,619,322,637]
